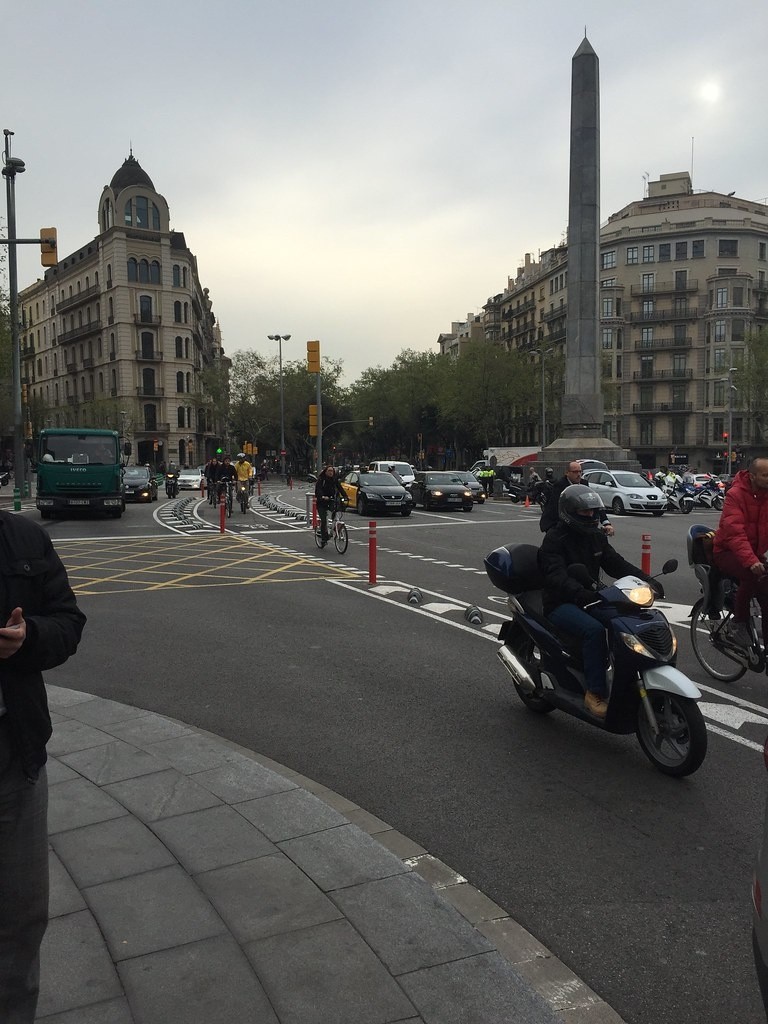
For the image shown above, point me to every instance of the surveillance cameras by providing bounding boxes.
[732,386,737,391]
[730,368,738,370]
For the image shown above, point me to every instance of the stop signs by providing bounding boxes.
[280,450,287,456]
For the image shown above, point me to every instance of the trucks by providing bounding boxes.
[470,447,542,478]
[24,427,132,518]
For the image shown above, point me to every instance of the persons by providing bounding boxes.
[539,461,615,538]
[204,453,253,513]
[94,443,113,457]
[535,484,665,717]
[131,460,190,494]
[315,465,352,545]
[1,508,86,1022]
[712,457,768,648]
[478,467,557,505]
[652,464,693,512]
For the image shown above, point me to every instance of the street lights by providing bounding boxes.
[529,347,554,450]
[728,367,738,474]
[267,335,291,481]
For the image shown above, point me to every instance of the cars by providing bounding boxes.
[690,473,725,493]
[121,466,160,503]
[338,470,416,517]
[752,805,768,971]
[408,471,474,512]
[579,470,669,517]
[446,471,486,504]
[176,469,207,491]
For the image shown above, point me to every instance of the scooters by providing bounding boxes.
[483,542,709,778]
[163,470,181,499]
[306,470,324,483]
[0,446,15,488]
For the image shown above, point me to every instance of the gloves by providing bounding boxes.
[591,590,606,608]
[647,578,664,600]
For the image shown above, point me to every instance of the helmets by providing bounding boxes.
[236,453,246,460]
[545,467,553,476]
[170,463,175,467]
[558,484,603,529]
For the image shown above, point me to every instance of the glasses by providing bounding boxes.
[569,470,583,474]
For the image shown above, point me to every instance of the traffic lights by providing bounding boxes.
[724,451,728,462]
[215,438,222,455]
[723,432,728,443]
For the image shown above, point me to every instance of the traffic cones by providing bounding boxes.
[524,495,530,507]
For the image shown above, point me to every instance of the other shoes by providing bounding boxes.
[321,540,327,545]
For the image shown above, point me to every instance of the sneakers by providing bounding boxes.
[585,689,607,717]
[728,617,753,647]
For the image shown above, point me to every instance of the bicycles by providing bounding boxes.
[314,497,352,554]
[726,561,727,563]
[687,564,768,683]
[204,461,294,518]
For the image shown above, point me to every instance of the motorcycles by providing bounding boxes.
[508,475,540,503]
[650,472,727,514]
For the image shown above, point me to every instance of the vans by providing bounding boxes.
[368,460,416,492]
